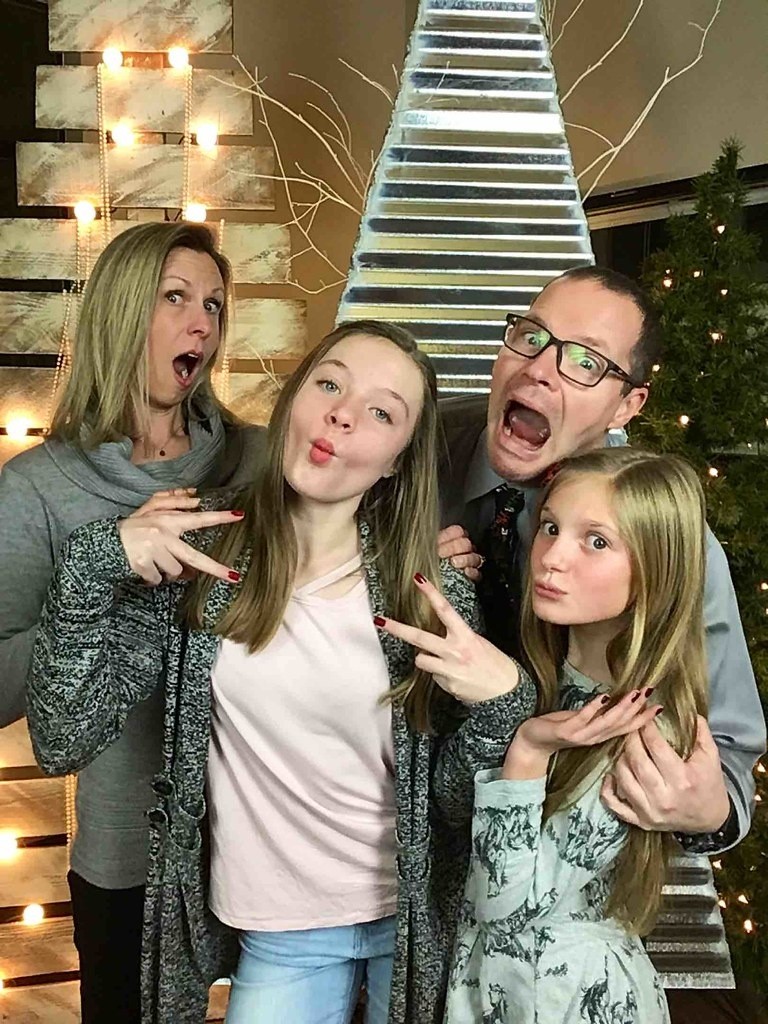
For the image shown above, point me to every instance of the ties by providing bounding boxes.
[474,483,523,661]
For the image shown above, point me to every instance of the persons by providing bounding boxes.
[0,218,767,1024]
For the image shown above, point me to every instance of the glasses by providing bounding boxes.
[503,313,642,387]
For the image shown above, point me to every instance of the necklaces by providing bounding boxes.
[152,424,186,456]
